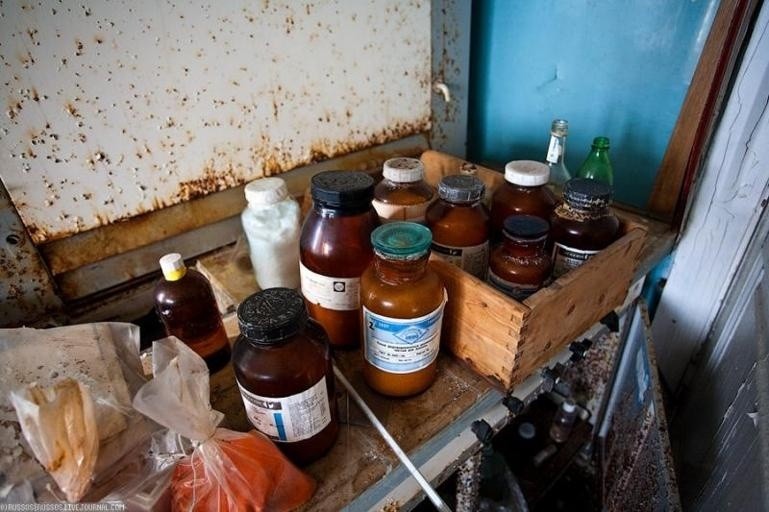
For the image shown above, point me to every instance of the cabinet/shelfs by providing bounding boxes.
[409,297,684,512]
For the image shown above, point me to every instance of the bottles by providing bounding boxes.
[298,169,381,348]
[425,175,489,279]
[486,216,551,304]
[232,287,337,462]
[370,157,439,224]
[240,177,303,290]
[358,223,448,398]
[548,119,575,191]
[154,253,231,373]
[549,398,577,445]
[571,136,616,204]
[489,159,551,243]
[519,423,536,444]
[545,179,621,281]
